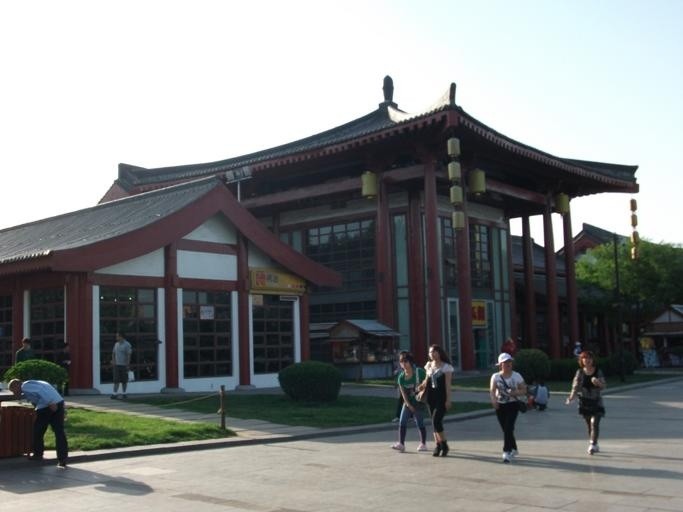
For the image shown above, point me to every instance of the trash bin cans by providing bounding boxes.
[0,406,39,458]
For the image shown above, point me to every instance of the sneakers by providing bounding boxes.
[588,443,599,455]
[29,456,66,466]
[391,443,404,451]
[503,448,519,463]
[417,443,427,451]
[111,394,128,399]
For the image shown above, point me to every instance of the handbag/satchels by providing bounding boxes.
[414,384,428,403]
[518,396,529,413]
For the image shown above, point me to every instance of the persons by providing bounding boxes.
[390,351,426,452]
[489,352,528,463]
[529,382,551,410]
[7,378,70,467]
[392,350,414,422]
[111,331,132,401]
[418,344,453,456]
[566,350,609,454]
[17,337,33,363]
[502,338,517,354]
[55,343,74,396]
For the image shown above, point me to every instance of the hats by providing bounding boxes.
[497,353,513,366]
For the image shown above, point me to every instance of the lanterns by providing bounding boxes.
[470,167,487,197]
[557,192,569,216]
[630,199,640,260]
[447,134,465,232]
[360,170,378,200]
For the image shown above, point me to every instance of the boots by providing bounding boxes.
[432,440,449,457]
